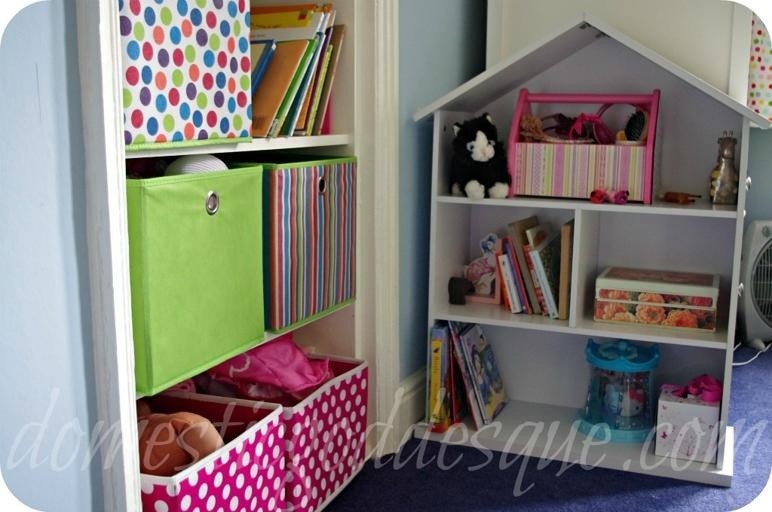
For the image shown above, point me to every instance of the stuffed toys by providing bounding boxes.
[447,112,512,198]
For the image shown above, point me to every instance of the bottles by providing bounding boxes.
[709,139,739,205]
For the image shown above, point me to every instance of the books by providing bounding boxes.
[426,321,511,434]
[249,2,347,136]
[498,213,575,322]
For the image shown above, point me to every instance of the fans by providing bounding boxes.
[744,219,772,344]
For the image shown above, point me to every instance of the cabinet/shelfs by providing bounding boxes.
[75,1,398,512]
[412,12,772,487]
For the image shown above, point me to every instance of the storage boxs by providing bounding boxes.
[655,384,719,464]
[136,393,286,511]
[119,0,252,153]
[594,265,722,333]
[260,154,357,336]
[127,166,265,396]
[508,88,660,206]
[284,351,368,510]
